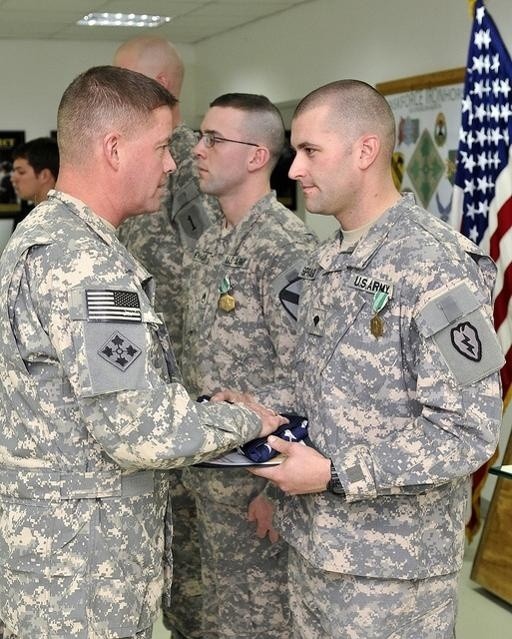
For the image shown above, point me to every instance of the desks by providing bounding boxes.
[470,465,512,608]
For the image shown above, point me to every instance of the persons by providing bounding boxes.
[113,35,225,639]
[208,75,508,639]
[2,64,289,639]
[179,92,318,639]
[9,136,61,208]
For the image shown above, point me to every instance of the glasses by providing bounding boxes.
[193,130,261,152]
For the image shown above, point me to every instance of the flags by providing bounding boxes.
[444,0,511,546]
